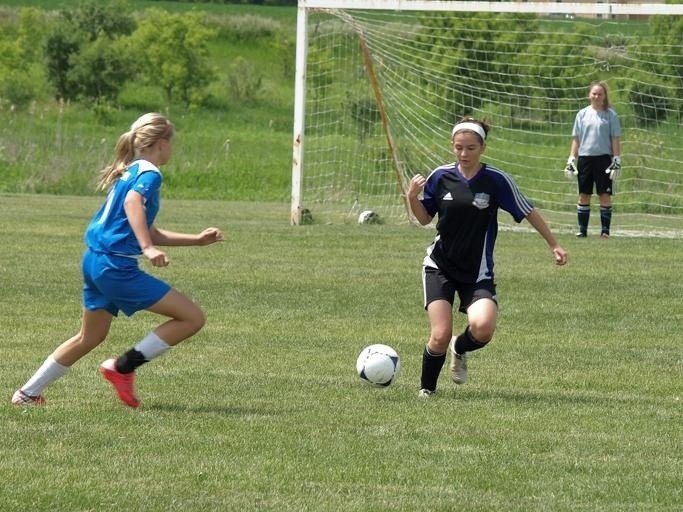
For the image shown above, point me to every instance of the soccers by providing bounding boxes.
[354,344,400,388]
[359,211,382,224]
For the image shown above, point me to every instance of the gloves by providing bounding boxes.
[605,156,621,182]
[564,157,579,179]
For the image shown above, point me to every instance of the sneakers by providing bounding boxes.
[449,336,467,384]
[100,358,141,407]
[576,232,609,239]
[11,389,46,405]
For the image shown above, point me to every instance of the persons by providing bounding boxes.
[406,116,567,399]
[9,112,228,408]
[564,83,621,238]
